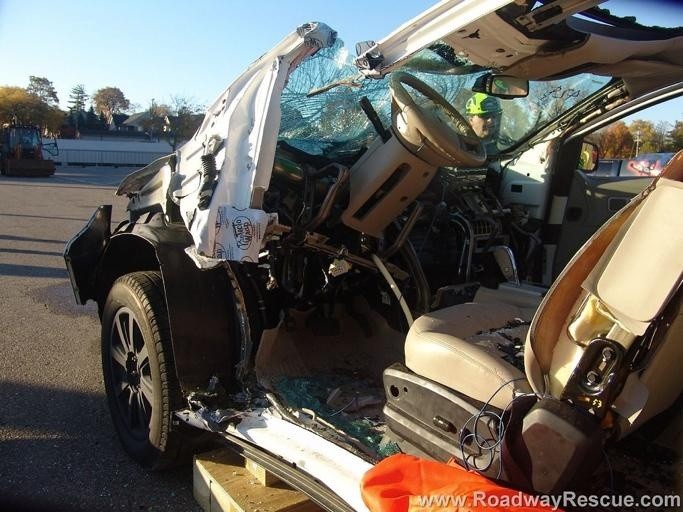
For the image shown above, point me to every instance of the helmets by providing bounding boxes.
[465,93,501,115]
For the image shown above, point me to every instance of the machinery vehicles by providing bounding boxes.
[0,123,56,176]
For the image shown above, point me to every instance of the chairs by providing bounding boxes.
[382,148,683,498]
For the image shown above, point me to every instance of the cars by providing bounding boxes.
[63,0,683,512]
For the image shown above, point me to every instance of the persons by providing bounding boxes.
[465,92,518,159]
